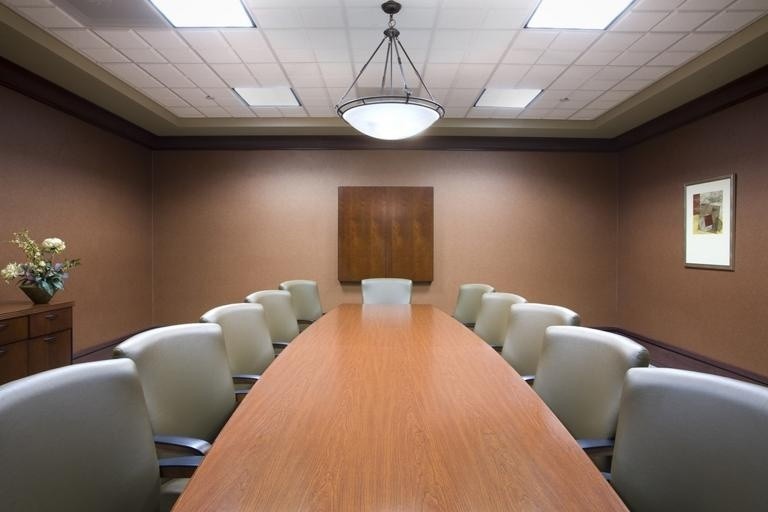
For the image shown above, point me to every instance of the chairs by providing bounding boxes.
[114,323,252,478]
[451,284,495,331]
[501,303,581,387]
[279,279,325,330]
[600,368,767,512]
[0,358,206,512]
[200,302,279,390]
[532,325,650,472]
[472,292,528,354]
[360,278,413,304]
[244,290,300,354]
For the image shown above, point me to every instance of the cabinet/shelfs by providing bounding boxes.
[0,299,75,386]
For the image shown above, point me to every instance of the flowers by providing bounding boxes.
[1,229,82,295]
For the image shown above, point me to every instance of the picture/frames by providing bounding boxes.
[686,174,737,272]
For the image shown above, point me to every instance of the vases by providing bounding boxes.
[19,286,60,304]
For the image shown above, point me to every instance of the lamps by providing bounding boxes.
[336,1,446,141]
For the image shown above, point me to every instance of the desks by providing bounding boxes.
[171,303,631,507]
